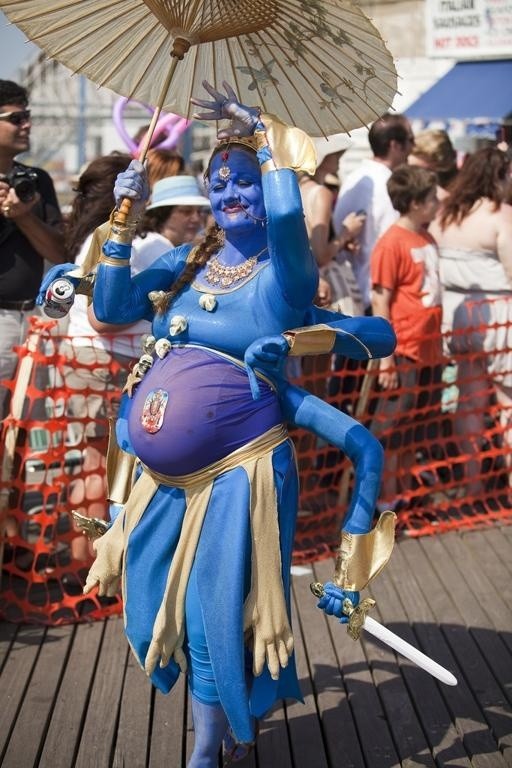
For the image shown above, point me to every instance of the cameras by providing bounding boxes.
[0,165,41,203]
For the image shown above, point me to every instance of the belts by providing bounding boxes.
[0,299,35,311]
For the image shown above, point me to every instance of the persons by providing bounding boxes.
[94,78,321,766]
[0,77,69,422]
[56,114,510,606]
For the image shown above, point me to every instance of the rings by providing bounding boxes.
[221,98,227,105]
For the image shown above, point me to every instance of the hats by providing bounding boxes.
[144,174,212,211]
[312,134,352,167]
[408,129,457,171]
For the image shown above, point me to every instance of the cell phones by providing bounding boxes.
[356,210,366,216]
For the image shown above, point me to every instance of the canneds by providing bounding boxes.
[43,278,75,318]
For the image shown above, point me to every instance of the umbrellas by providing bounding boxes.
[0,0,403,226]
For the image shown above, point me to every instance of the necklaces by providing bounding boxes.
[204,245,267,290]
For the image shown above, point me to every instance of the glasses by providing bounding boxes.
[0,110,30,123]
